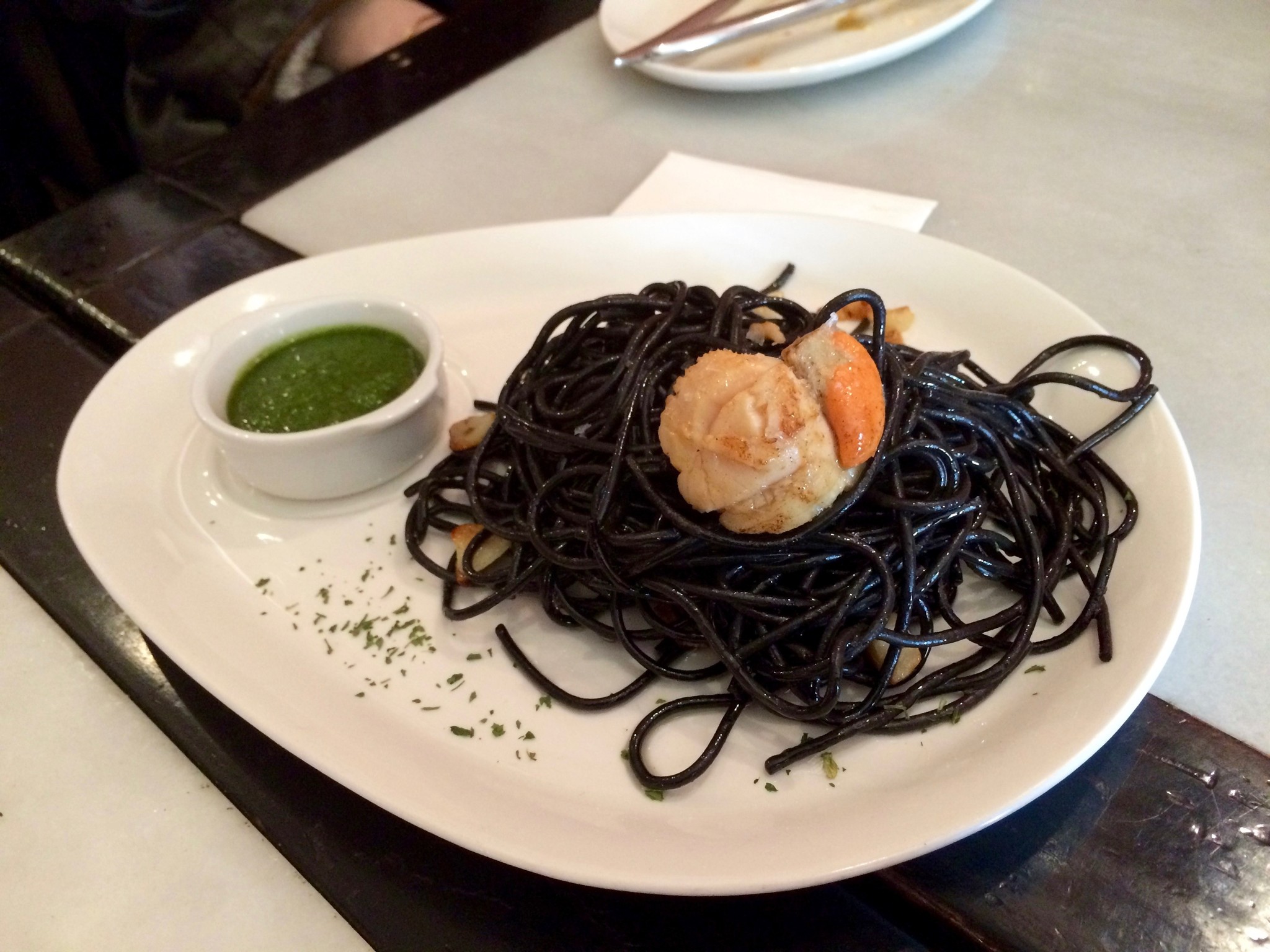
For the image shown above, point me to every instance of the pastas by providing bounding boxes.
[398,260,1160,795]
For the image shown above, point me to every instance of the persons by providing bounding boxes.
[183,0,452,108]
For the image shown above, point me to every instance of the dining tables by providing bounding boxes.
[0,0,1269,952]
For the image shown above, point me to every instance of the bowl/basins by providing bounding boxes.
[188,294,449,503]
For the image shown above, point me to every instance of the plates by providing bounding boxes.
[598,0,995,92]
[52,216,1198,895]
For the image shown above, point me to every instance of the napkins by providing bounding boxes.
[604,152,940,237]
